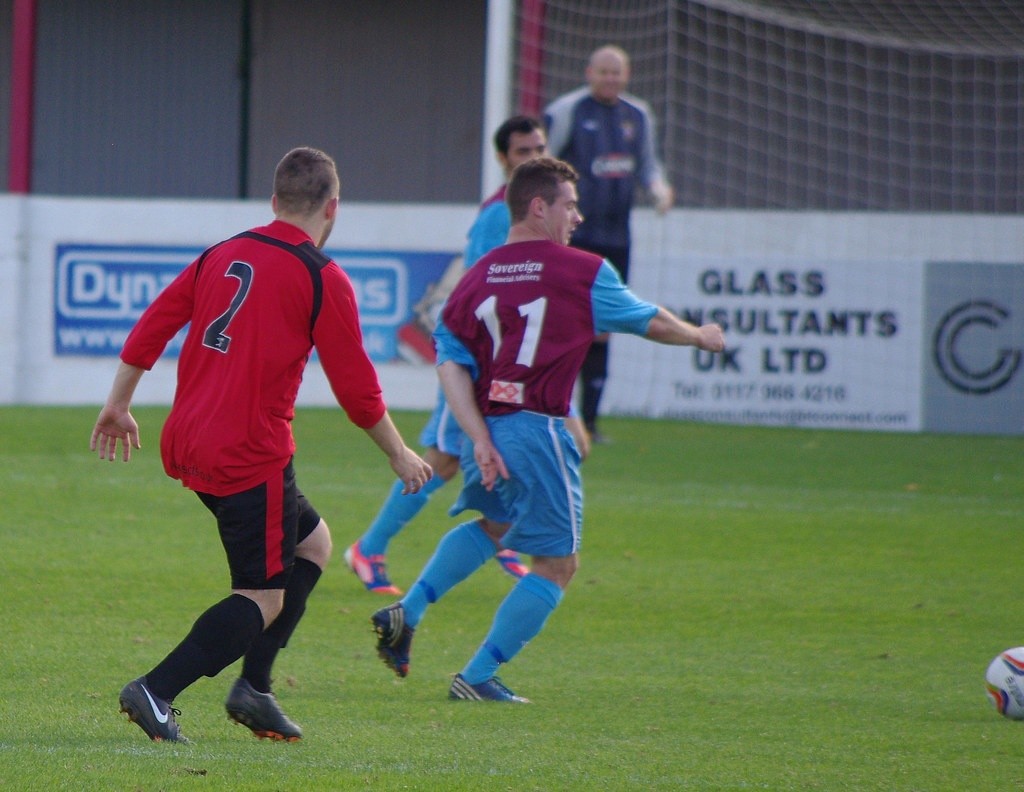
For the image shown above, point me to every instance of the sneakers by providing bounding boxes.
[370,602,415,676]
[496,547,530,578]
[343,539,404,596]
[449,674,534,707]
[118,677,188,743]
[224,676,304,742]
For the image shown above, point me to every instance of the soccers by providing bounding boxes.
[985,646,1024,720]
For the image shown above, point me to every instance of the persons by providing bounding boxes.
[372,158,724,704]
[91,147,433,745]
[479,117,553,205]
[344,203,591,597]
[545,44,675,442]
